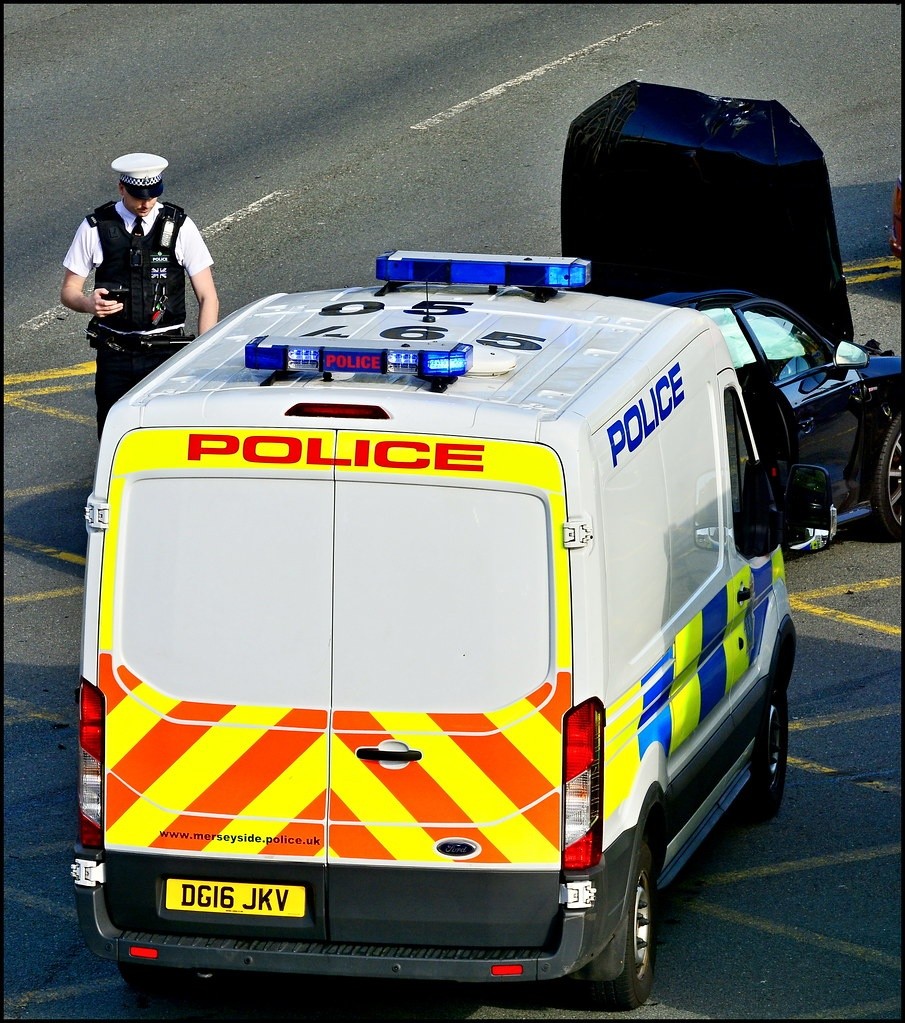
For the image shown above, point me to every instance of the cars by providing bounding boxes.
[642,287,902,548]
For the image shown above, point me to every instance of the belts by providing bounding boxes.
[89,325,182,349]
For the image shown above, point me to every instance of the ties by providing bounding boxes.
[132,217,144,236]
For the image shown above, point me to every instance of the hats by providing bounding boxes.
[111,152,169,199]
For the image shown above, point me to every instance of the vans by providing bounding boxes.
[66,248,843,1012]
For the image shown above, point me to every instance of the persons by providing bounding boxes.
[61,152,220,445]
